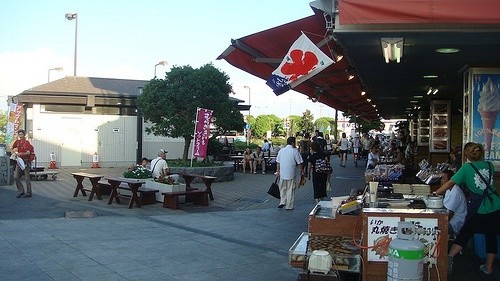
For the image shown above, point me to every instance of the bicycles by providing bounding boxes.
[352,145,362,168]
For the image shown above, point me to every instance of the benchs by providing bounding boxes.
[97,181,159,205]
[232,163,277,173]
[161,190,210,209]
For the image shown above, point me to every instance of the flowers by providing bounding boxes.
[151,172,180,185]
[122,163,152,179]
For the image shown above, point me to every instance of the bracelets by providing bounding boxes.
[433,192,438,196]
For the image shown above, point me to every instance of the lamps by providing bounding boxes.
[329,47,344,62]
[344,67,355,80]
[381,37,404,64]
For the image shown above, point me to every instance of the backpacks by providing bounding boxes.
[18,140,35,161]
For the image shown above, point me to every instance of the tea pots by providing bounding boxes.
[421,192,443,208]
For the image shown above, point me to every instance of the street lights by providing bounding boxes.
[48,67,64,83]
[65,12,78,75]
[155,60,169,77]
[244,85,251,121]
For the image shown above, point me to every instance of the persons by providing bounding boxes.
[352,134,363,162]
[242,146,254,174]
[307,143,331,204]
[11,129,35,198]
[392,147,407,179]
[299,133,312,177]
[311,129,332,166]
[253,147,265,174]
[441,170,467,251]
[262,139,269,152]
[150,148,179,184]
[359,134,400,160]
[276,137,303,210]
[366,146,381,172]
[337,133,348,167]
[432,142,500,275]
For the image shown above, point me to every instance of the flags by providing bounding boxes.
[266,31,335,96]
[193,108,215,158]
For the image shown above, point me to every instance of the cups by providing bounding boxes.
[369,182,379,202]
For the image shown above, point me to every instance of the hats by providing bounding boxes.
[160,149,167,154]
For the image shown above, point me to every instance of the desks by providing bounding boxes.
[104,177,147,209]
[71,171,104,201]
[229,156,277,171]
[181,173,219,201]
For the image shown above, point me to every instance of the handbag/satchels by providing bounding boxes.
[325,173,333,191]
[267,175,281,199]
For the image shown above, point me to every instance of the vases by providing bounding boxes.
[118,176,147,198]
[145,178,187,204]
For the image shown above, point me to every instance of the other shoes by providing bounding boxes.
[447,255,454,268]
[16,192,25,197]
[480,264,492,275]
[262,172,265,175]
[23,192,32,198]
[278,204,285,208]
[286,208,294,211]
[254,171,257,174]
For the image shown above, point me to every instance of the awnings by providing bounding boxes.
[217,8,381,123]
